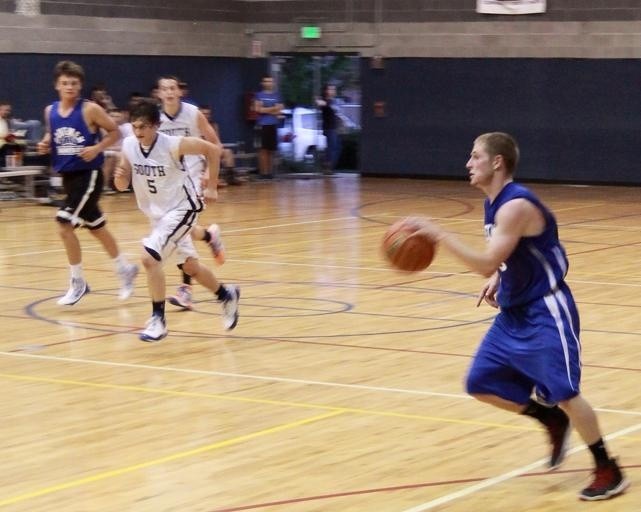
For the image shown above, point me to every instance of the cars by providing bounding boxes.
[277,109,360,162]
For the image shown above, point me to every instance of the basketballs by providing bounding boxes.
[383,219,436,272]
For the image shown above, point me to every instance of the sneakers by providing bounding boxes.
[580,458,630,501]
[166,285,192,311]
[57,277,89,307]
[544,406,573,471]
[207,224,225,264]
[221,287,242,331]
[118,263,139,303]
[141,317,168,341]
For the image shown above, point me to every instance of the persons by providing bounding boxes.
[37,63,137,305]
[99,109,131,195]
[395,133,629,502]
[126,93,145,109]
[313,84,344,175]
[88,85,117,111]
[196,104,245,188]
[176,80,195,106]
[152,77,225,308]
[114,104,240,341]
[250,75,284,182]
[0,100,50,183]
